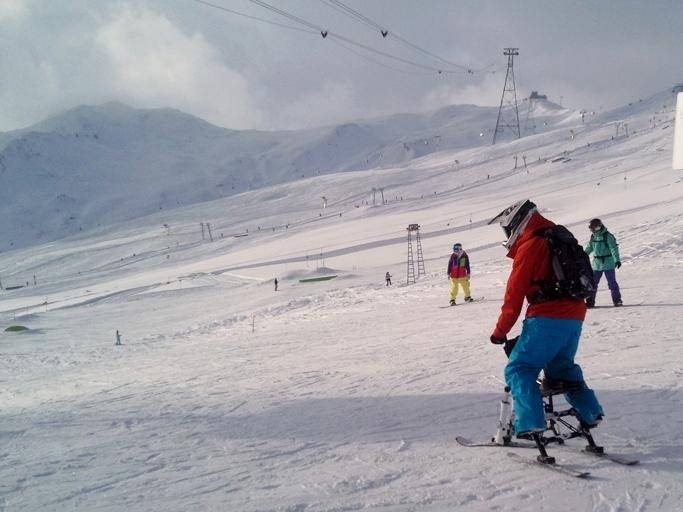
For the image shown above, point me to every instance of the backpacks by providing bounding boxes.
[526,225,597,303]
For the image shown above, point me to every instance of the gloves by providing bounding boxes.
[614,261,621,269]
[467,275,470,280]
[490,334,507,344]
[448,276,450,280]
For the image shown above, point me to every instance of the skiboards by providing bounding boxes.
[454,421,548,448]
[502,440,640,479]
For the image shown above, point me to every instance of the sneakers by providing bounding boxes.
[585,299,595,308]
[595,413,605,423]
[614,300,623,307]
[464,296,473,302]
[450,299,456,306]
[517,427,545,436]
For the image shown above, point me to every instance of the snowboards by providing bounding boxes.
[585,301,645,309]
[438,296,484,308]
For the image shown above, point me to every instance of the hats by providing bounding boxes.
[588,218,602,228]
[454,243,461,250]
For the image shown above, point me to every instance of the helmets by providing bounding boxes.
[488,198,537,242]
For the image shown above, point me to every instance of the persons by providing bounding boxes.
[386,271,393,286]
[116,330,122,345]
[583,219,623,305]
[488,199,604,444]
[446,243,475,306]
[274,277,278,292]
